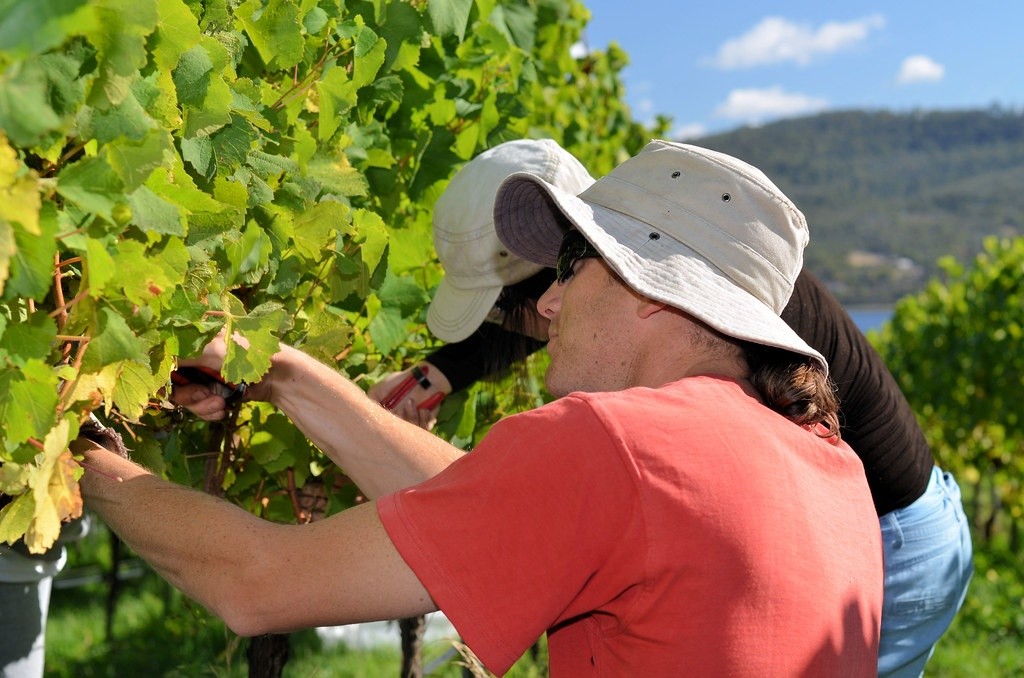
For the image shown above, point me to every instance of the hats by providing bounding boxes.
[425,141,598,342]
[495,140,830,387]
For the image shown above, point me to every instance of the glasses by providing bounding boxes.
[556,230,600,283]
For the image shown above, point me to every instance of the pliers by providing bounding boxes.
[169,359,246,407]
[379,364,446,417]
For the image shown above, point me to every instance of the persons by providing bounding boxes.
[69,139,885,678]
[366,137,972,678]
